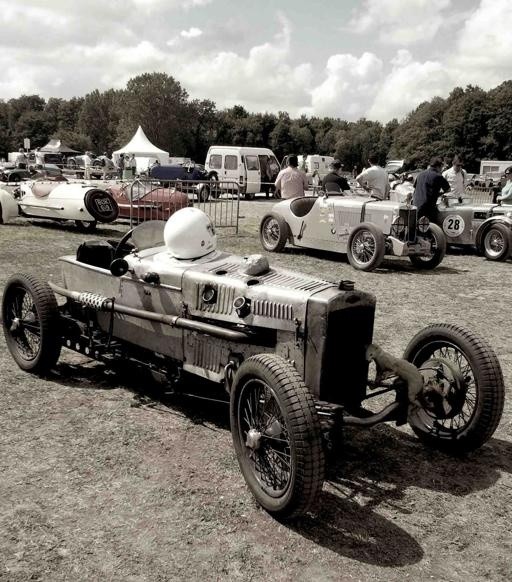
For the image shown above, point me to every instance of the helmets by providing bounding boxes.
[163,208,217,259]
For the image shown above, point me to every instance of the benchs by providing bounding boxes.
[290,196,319,216]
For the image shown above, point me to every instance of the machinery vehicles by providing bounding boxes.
[4,205,504,520]
[404,189,512,263]
[257,188,447,272]
[0,180,194,231]
[1,146,67,182]
[148,159,220,201]
[67,150,113,178]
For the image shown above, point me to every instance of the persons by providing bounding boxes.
[492,165,512,204]
[412,155,451,225]
[101,151,137,180]
[356,153,389,201]
[84,152,91,180]
[440,154,468,197]
[322,159,350,192]
[261,154,320,201]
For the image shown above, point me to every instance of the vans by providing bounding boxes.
[295,155,336,186]
[204,145,282,201]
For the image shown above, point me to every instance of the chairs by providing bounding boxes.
[77,240,135,274]
